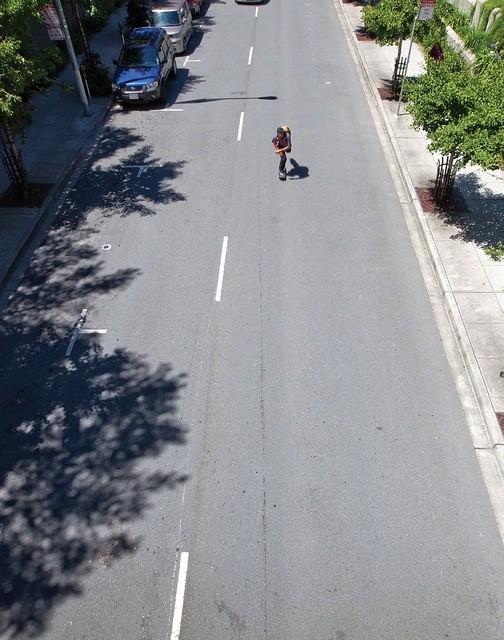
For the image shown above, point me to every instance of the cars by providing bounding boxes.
[187,0,203,15]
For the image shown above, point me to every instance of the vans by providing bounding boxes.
[144,0,194,54]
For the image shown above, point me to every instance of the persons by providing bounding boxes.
[272,127,291,180]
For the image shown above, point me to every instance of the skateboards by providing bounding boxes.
[278,167,286,180]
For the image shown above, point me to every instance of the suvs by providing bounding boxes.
[110,26,178,107]
